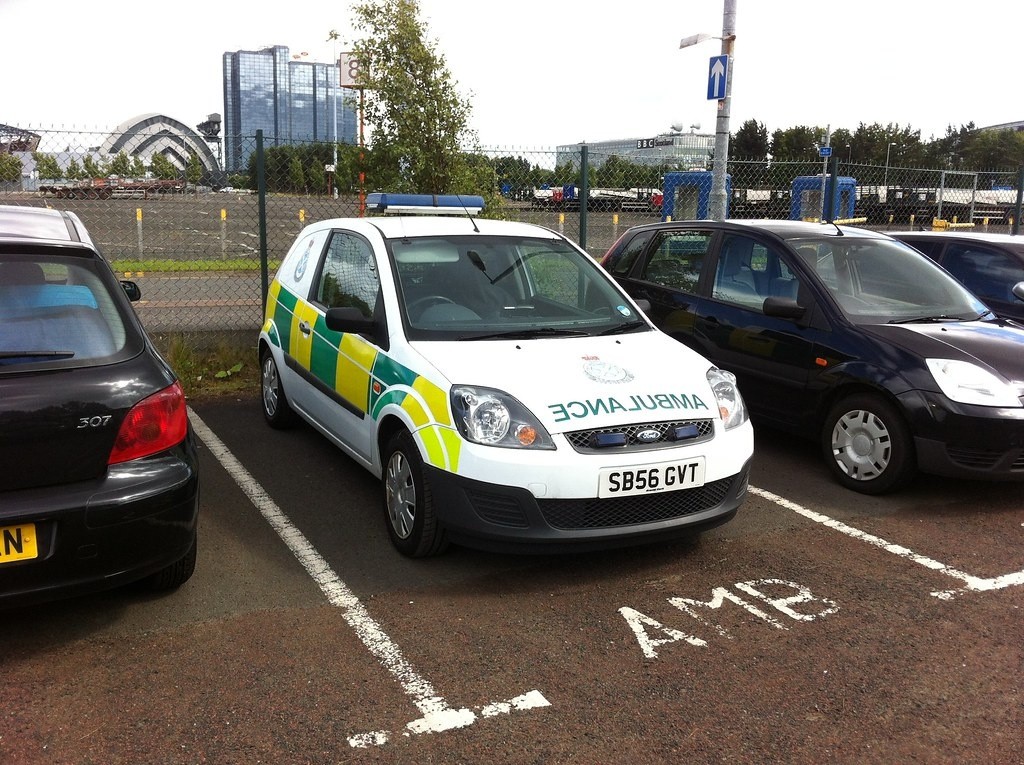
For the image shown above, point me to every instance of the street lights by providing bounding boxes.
[846,145,851,177]
[884,143,896,186]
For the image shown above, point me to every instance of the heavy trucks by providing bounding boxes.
[39,172,183,200]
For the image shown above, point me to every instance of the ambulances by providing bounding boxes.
[258,193,754,560]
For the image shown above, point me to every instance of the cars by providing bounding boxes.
[0,205,200,608]
[599,219,1024,494]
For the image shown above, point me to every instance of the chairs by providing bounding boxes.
[717,254,752,302]
[778,247,817,300]
[0,262,44,284]
[439,259,517,319]
